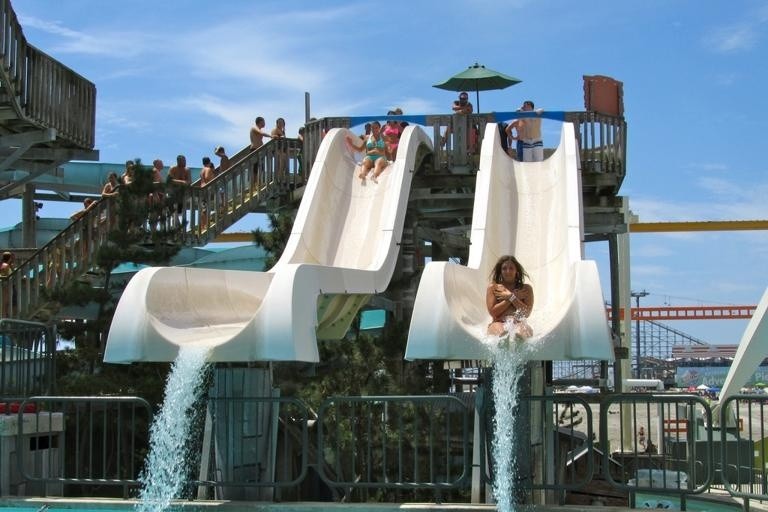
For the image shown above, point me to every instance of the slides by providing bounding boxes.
[103,124,434,368]
[404,120,616,361]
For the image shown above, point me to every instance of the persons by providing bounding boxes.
[0,251,11,319]
[485,254,535,343]
[636,426,646,450]
[440,91,512,155]
[270,118,288,176]
[9,253,17,316]
[504,108,524,161]
[213,145,229,215]
[249,117,278,192]
[346,107,411,181]
[518,100,543,161]
[199,157,213,229]
[296,126,304,175]
[47,154,192,289]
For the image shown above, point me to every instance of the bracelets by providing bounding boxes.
[508,294,515,302]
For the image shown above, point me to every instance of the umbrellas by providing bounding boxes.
[431,63,523,114]
[695,384,709,389]
[754,382,766,387]
